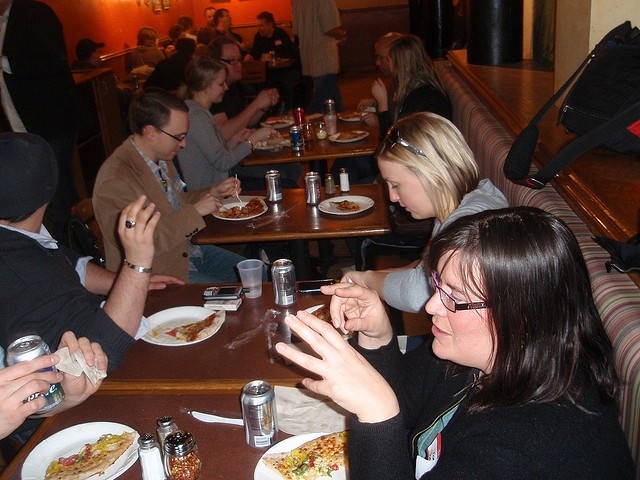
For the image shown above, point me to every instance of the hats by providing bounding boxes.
[0,131,59,221]
[75,38,104,57]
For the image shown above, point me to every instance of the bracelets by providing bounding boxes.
[123,259,152,273]
[245,139,253,149]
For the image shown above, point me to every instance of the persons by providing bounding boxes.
[70,38,132,116]
[451,0,468,50]
[0,331,109,441]
[92,90,267,284]
[356,32,404,126]
[331,35,453,184]
[156,37,195,69]
[252,11,299,112]
[130,5,241,41]
[0,0,80,206]
[339,111,509,314]
[292,0,348,115]
[0,132,186,373]
[124,27,167,72]
[175,58,278,193]
[207,39,304,187]
[274,206,639,480]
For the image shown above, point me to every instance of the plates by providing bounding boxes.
[318,195,375,216]
[254,141,284,151]
[140,306,222,347]
[294,304,353,340]
[338,112,369,122]
[21,422,140,480]
[254,432,350,480]
[281,139,292,147]
[329,131,370,143]
[213,202,268,221]
[261,120,295,129]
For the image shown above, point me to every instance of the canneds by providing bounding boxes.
[269,51,276,62]
[264,170,282,202]
[322,99,336,117]
[240,382,279,447]
[293,105,306,125]
[305,172,322,206]
[290,127,305,152]
[5,335,66,415]
[271,259,295,308]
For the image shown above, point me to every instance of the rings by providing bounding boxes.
[125,221,136,228]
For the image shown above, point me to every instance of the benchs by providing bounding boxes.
[368,62,640,479]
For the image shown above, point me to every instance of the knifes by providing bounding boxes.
[192,411,245,426]
[221,206,228,211]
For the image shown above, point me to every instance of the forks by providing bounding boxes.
[236,174,242,210]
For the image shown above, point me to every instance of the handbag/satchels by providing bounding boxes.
[503,20,639,190]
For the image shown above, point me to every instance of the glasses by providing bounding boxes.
[156,126,185,143]
[383,126,428,159]
[221,58,244,64]
[430,269,491,313]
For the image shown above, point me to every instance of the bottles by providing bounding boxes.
[339,168,351,194]
[325,174,336,197]
[302,123,315,144]
[315,122,327,140]
[323,99,337,136]
[156,416,180,456]
[163,430,202,480]
[138,432,168,480]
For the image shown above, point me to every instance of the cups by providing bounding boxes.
[236,259,264,298]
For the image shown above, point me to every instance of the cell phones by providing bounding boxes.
[297,278,335,293]
[203,285,250,300]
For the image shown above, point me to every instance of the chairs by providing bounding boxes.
[72,198,104,268]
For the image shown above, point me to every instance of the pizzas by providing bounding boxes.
[339,109,361,119]
[165,312,222,342]
[266,127,284,147]
[45,431,135,479]
[336,131,365,140]
[267,88,279,106]
[218,198,264,218]
[262,428,348,480]
